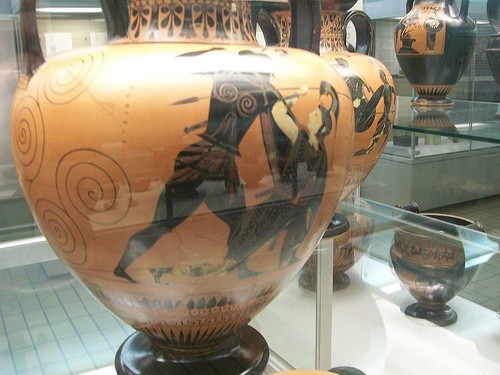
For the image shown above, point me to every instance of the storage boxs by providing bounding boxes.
[393,135,418,147]
